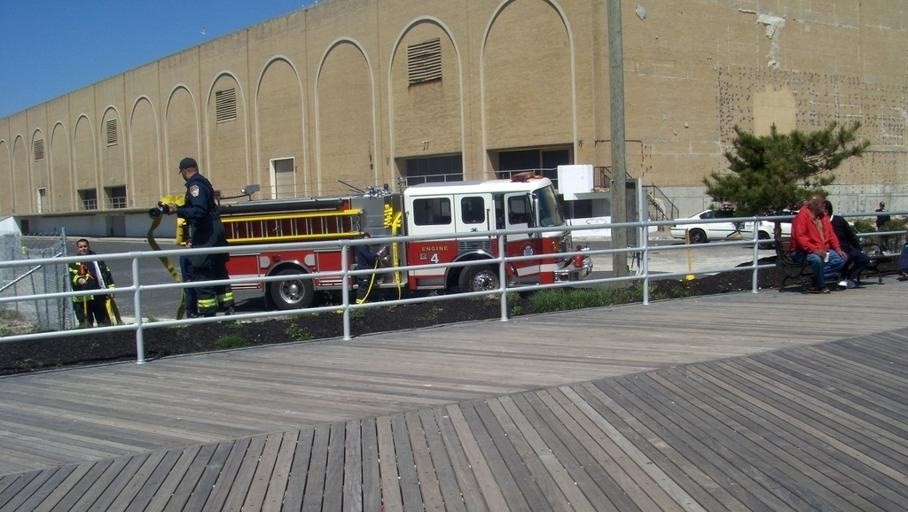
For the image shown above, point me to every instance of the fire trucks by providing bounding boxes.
[146,169,593,300]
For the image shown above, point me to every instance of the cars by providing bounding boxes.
[670,209,800,250]
[767,208,800,223]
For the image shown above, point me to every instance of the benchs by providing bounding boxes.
[773,238,902,293]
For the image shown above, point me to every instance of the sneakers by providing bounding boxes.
[820,279,855,294]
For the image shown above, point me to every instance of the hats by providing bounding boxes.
[178,158,197,174]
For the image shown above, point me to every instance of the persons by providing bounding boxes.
[167,157,237,316]
[67,238,114,326]
[790,194,848,294]
[353,233,388,303]
[175,197,198,318]
[874,200,891,228]
[824,198,871,288]
[896,242,908,281]
